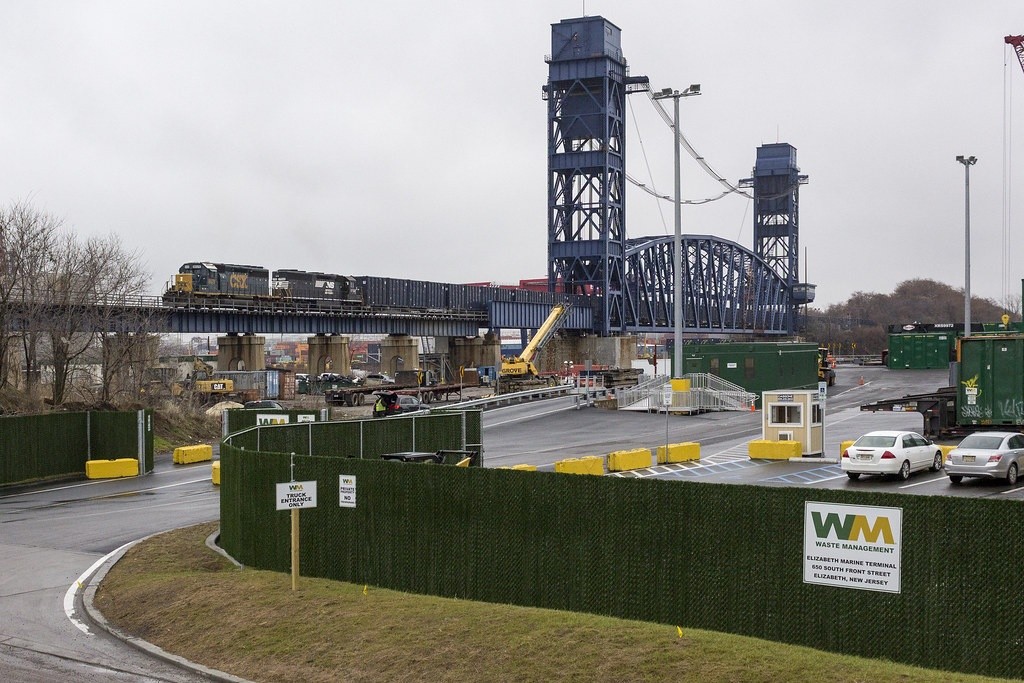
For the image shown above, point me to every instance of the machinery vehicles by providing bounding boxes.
[817,347,837,386]
[499,293,575,380]
[172,354,234,399]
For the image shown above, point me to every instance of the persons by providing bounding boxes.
[376,397,390,418]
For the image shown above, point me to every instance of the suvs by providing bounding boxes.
[371,389,430,419]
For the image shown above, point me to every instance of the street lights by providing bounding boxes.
[954,154,979,336]
[654,80,704,377]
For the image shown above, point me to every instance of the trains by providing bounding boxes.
[159,262,875,333]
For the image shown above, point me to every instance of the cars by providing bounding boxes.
[361,373,396,384]
[318,373,345,389]
[293,374,316,392]
[840,431,942,483]
[943,432,1024,485]
[245,400,284,410]
